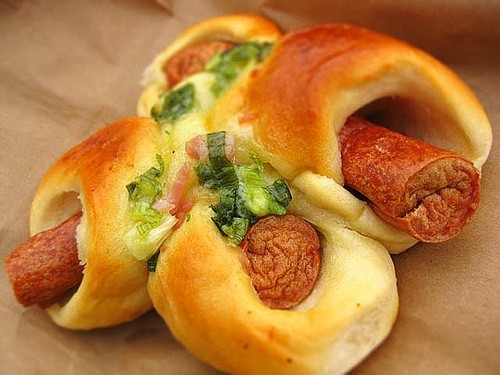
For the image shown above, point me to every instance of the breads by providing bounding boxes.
[19,8,492,374]
